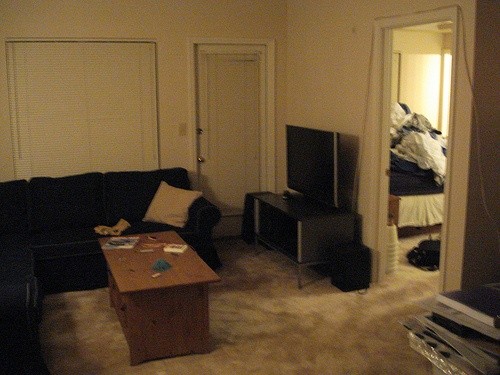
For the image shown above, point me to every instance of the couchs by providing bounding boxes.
[0,166,221,375]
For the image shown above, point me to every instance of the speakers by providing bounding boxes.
[332,241,371,292]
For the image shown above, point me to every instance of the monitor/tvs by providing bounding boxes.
[285,124,339,208]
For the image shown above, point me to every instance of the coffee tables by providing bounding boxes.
[99,231,222,366]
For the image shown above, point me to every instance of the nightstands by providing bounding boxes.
[389,195,402,227]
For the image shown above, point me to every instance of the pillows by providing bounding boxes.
[142,181,202,228]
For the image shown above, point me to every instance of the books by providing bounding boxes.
[407,282,500,375]
[101,236,140,250]
[163,243,188,254]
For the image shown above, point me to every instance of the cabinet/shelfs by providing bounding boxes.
[253,193,362,290]
